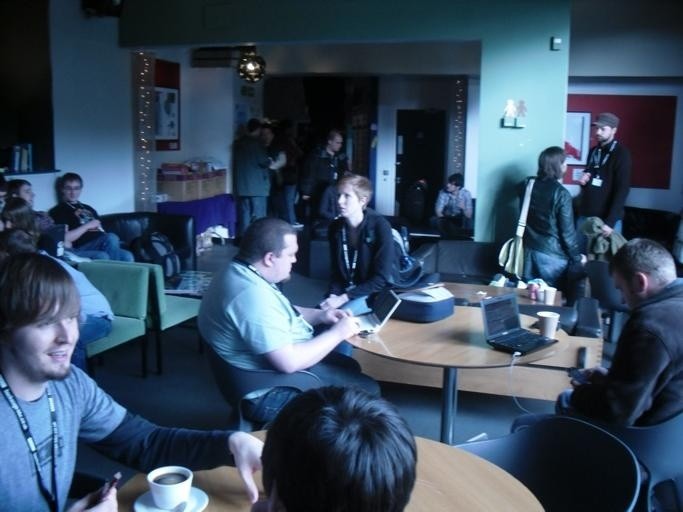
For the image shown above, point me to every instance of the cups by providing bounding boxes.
[578,167,592,186]
[145,464,193,508]
[527,281,557,306]
[536,311,559,340]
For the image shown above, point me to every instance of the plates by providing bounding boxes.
[132,485,208,512]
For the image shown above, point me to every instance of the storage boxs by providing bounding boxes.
[156,169,226,202]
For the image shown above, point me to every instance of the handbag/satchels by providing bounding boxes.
[497,233,526,282]
[395,274,455,324]
[137,230,181,279]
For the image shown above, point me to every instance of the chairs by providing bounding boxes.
[202,340,324,431]
[597,411,683,512]
[461,198,478,242]
[453,415,641,512]
[77,260,203,379]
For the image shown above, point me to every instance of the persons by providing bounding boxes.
[314,173,400,362]
[486,146,588,290]
[2,230,114,380]
[574,111,633,273]
[1,179,101,264]
[1,254,272,511]
[512,239,682,478]
[48,173,134,262]
[428,174,475,242]
[232,118,343,241]
[262,386,418,512]
[195,218,381,428]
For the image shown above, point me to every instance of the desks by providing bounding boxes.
[157,194,237,246]
[341,283,570,446]
[164,270,214,297]
[120,429,545,512]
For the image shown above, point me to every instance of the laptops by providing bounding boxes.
[479,292,558,356]
[354,289,402,334]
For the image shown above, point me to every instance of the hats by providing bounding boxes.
[591,112,619,127]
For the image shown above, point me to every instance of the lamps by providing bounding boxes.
[236,46,266,83]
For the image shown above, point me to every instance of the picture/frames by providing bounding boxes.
[565,113,591,165]
[156,87,179,141]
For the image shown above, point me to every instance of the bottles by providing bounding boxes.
[13,142,34,171]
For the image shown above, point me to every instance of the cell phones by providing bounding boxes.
[566,367,588,384]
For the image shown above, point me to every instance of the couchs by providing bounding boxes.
[410,240,603,338]
[99,212,197,276]
